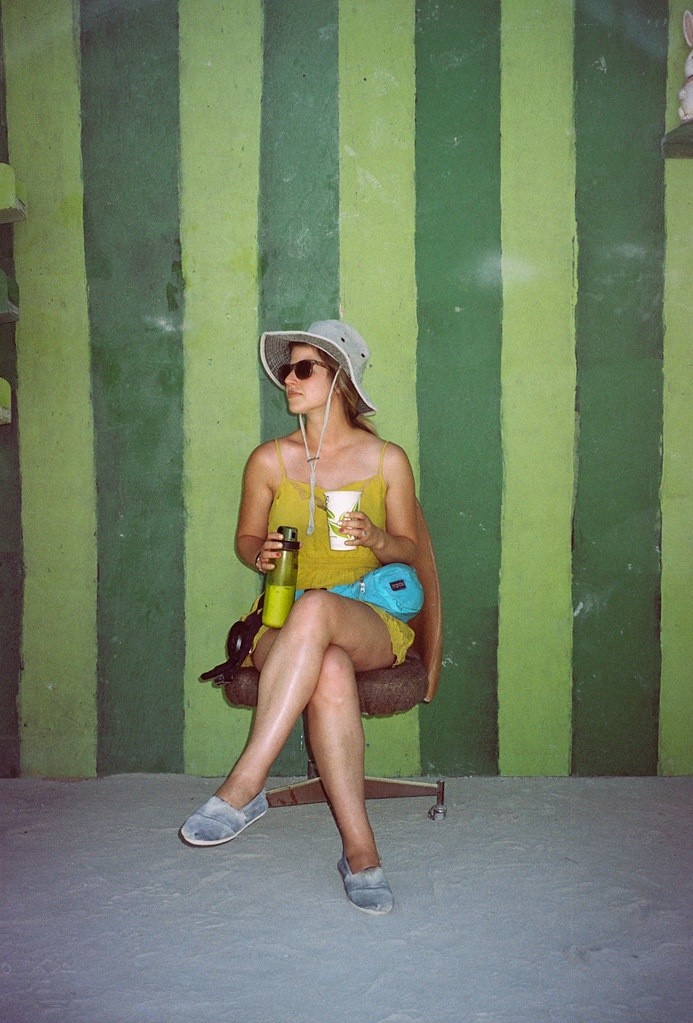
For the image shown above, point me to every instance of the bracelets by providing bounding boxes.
[255,550,267,575]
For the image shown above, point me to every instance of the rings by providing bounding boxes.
[361,530,366,538]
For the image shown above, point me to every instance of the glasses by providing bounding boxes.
[278,359,334,380]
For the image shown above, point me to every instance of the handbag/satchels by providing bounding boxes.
[294,562,424,623]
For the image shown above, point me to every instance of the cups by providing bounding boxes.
[323,491,362,551]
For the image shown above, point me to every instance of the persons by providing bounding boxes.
[180,319,419,915]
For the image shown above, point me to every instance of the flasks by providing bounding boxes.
[263,526,300,628]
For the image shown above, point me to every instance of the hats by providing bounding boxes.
[260,320,377,416]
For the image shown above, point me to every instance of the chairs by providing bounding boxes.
[225,498,447,824]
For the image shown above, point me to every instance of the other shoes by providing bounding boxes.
[181,786,268,846]
[337,848,394,915]
